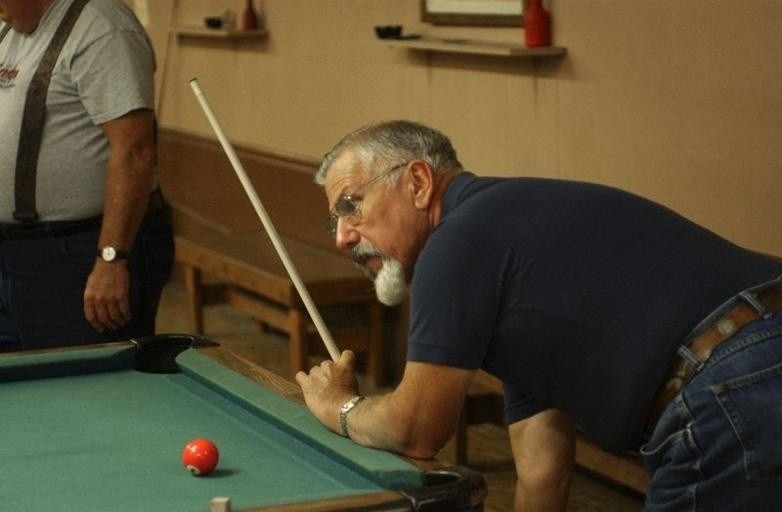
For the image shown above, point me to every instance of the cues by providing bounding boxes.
[188,74,341,364]
[154,0,175,123]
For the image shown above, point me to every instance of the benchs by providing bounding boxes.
[170,228,393,394]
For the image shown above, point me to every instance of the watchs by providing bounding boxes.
[95,246,133,263]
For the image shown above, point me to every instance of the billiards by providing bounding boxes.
[182,438,218,475]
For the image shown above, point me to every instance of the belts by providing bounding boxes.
[642,284,782,433]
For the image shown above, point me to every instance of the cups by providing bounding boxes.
[204,16,224,29]
[374,24,400,39]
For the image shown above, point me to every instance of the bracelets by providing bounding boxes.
[339,394,366,439]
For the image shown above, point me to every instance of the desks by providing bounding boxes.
[1,327,488,511]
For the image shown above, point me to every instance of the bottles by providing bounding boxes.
[243,0,258,31]
[524,0,552,48]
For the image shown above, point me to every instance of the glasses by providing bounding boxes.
[323,162,408,241]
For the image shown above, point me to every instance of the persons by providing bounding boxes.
[0,1,174,352]
[295,119,782,512]
